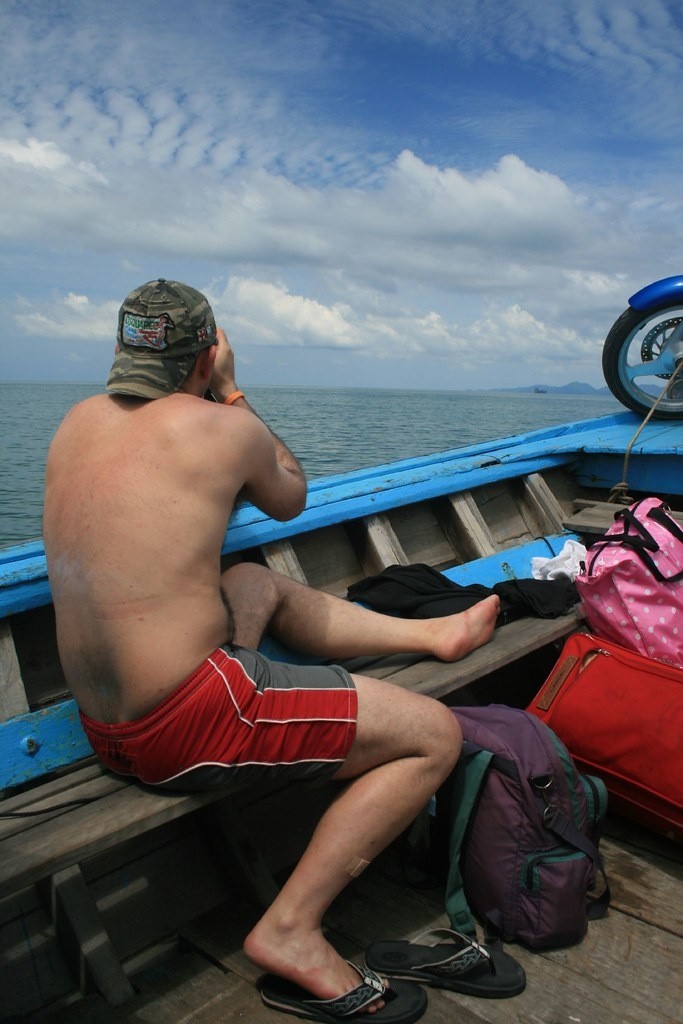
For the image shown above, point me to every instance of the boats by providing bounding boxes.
[534,388,548,394]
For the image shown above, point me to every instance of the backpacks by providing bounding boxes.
[410,703,610,952]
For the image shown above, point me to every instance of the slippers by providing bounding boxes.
[365,928,526,999]
[258,960,427,1024]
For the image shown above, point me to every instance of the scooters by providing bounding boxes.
[602,275,683,421]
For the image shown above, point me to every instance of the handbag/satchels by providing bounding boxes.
[574,496,683,669]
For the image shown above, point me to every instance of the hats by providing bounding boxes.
[106,278,217,399]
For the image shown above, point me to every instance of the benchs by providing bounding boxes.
[0,616,580,1009]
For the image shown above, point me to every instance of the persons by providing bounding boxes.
[43,279,501,1024]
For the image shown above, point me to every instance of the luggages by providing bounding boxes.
[525,633,683,846]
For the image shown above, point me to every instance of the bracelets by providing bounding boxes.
[224,390,245,405]
